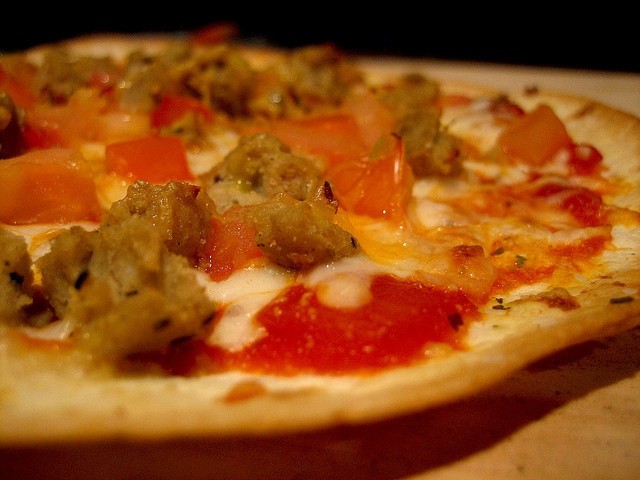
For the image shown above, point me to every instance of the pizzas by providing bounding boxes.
[0,37,640,443]
